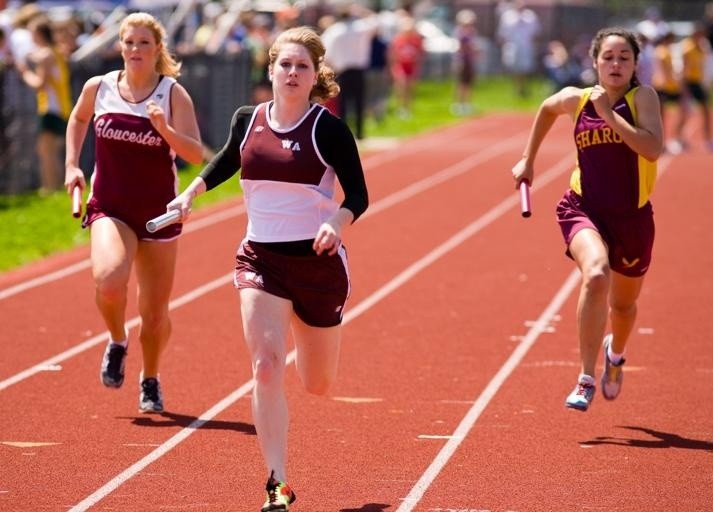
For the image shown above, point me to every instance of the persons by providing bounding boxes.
[64,13,203,416]
[630,1,713,153]
[514,27,664,412]
[2,1,596,198]
[165,25,368,512]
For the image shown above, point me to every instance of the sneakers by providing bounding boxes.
[100,342,128,389]
[140,373,163,414]
[601,334,626,400]
[565,383,594,411]
[260,477,296,511]
